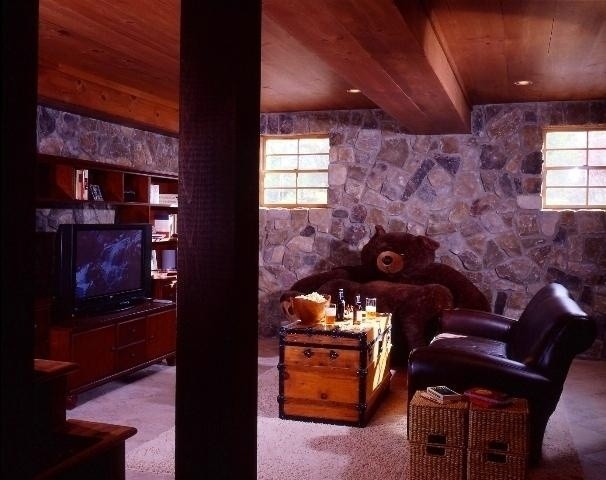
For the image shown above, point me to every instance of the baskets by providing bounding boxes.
[409,390,530,480]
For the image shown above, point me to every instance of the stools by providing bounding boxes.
[408,389,529,479]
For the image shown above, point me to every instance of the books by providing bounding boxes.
[81,168,89,201]
[76,168,80,200]
[463,386,515,404]
[461,395,495,408]
[150,183,176,242]
[426,385,461,400]
[420,392,458,404]
[88,184,104,202]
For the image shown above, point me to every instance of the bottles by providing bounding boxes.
[335,288,345,322]
[353,294,363,325]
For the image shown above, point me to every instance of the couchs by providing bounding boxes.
[278,222,490,363]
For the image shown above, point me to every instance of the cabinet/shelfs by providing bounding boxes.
[33,357,80,421]
[33,417,138,480]
[45,161,178,297]
[48,300,176,410]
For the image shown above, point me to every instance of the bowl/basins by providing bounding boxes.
[290,295,331,325]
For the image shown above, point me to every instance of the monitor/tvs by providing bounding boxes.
[59,223,152,315]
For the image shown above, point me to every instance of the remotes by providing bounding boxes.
[152,299,173,304]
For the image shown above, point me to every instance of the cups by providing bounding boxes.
[325,304,336,324]
[365,297,377,319]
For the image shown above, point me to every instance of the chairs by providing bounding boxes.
[405,281,594,442]
